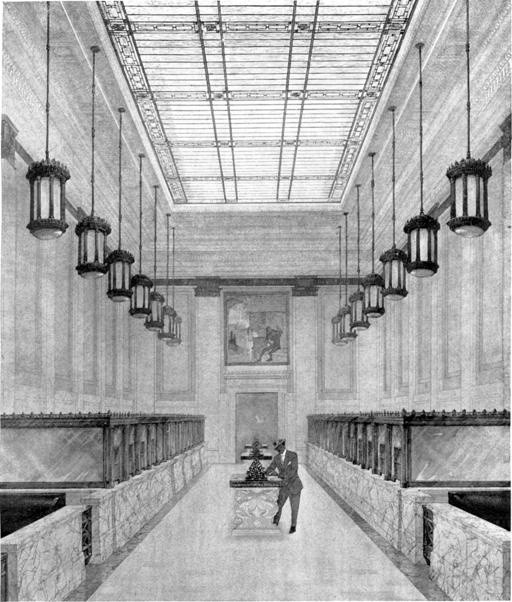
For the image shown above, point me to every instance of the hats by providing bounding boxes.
[273,438,286,450]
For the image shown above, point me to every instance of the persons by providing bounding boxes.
[258,319,282,363]
[266,440,303,534]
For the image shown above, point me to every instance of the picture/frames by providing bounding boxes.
[220,282,290,368]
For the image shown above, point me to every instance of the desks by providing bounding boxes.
[240,442,272,473]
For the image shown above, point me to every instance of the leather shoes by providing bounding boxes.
[289,526,295,533]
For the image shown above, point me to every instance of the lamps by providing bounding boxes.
[445,2,493,239]
[75,44,112,279]
[402,40,440,279]
[328,151,384,345]
[379,106,408,302]
[25,1,71,241]
[129,152,182,347]
[104,106,134,304]
